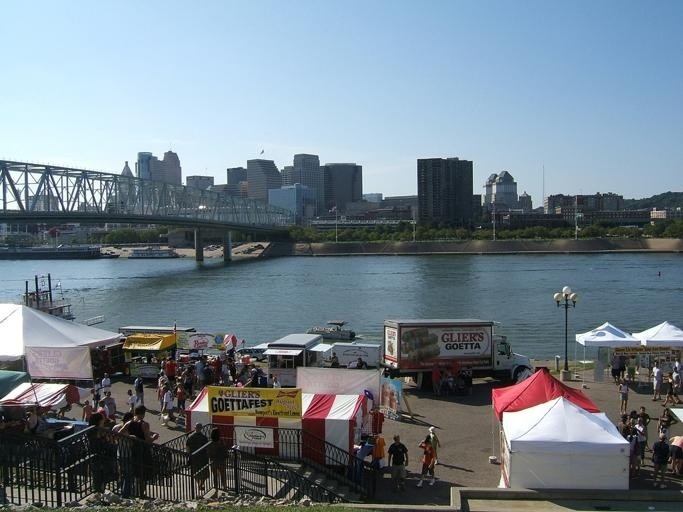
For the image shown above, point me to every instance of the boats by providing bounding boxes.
[125,247,186,258]
[304,318,356,341]
[52,245,120,259]
[18,273,104,326]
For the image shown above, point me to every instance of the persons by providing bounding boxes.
[330,352,339,368]
[610,349,683,481]
[387,434,409,488]
[429,426,440,465]
[416,436,436,488]
[432,360,459,397]
[82,346,281,500]
[27,407,39,435]
[356,358,367,370]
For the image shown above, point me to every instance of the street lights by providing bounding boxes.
[553,283,579,381]
[333,206,338,243]
[410,208,416,242]
[570,193,578,240]
[491,202,496,241]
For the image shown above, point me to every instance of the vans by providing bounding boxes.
[235,347,267,361]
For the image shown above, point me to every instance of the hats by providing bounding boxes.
[429,427,435,433]
[135,405,148,414]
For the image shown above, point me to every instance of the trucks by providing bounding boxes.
[381,318,534,396]
[116,324,196,336]
[330,340,382,369]
